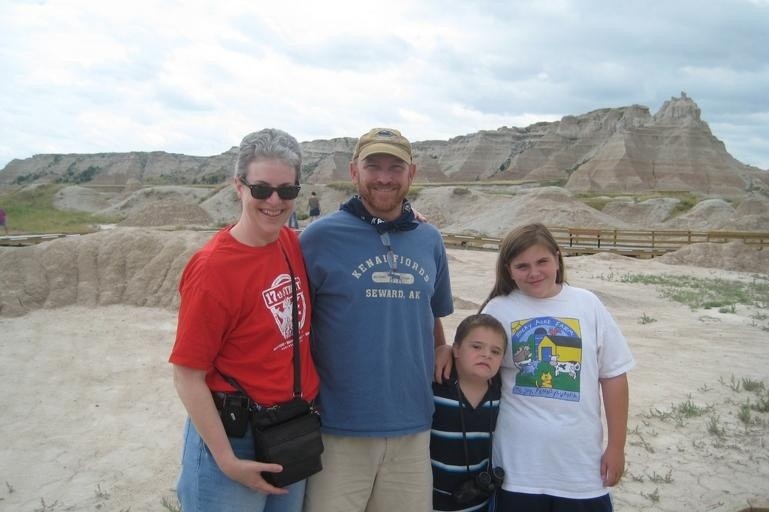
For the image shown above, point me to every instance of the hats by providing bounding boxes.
[353,127,413,166]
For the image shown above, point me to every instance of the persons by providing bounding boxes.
[434,223,637,511]
[0,207,9,235]
[288,213,299,229]
[290,126,455,510]
[304,191,320,222]
[433,314,509,510]
[168,129,428,510]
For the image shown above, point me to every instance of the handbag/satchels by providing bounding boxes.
[212,391,248,437]
[247,397,325,489]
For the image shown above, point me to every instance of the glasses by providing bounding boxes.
[238,175,302,199]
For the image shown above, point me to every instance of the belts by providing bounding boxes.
[211,392,249,412]
